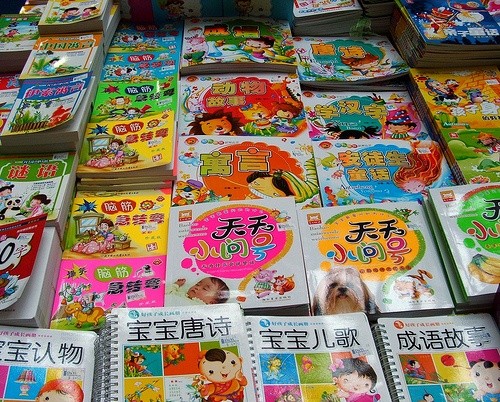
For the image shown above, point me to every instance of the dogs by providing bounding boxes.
[310,266,382,315]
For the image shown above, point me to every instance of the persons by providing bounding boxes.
[164,277,231,307]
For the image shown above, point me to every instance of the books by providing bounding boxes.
[0,0,500,402]
